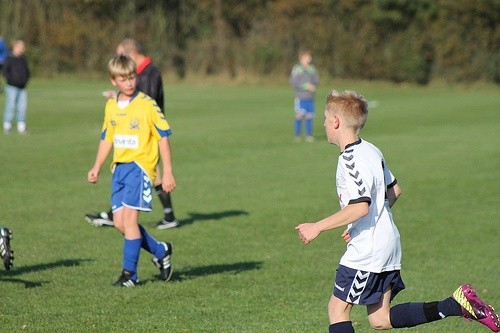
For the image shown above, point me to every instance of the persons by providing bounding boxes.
[296,90,500,333]
[0,38,9,91]
[3,39,30,132]
[289,47,320,142]
[84,39,180,230]
[87,53,177,288]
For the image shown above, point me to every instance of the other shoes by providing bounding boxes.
[0,228,15,272]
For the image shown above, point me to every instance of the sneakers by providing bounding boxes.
[113,269,141,287]
[156,218,180,230]
[151,240,173,282]
[84,212,114,227]
[452,284,500,332]
